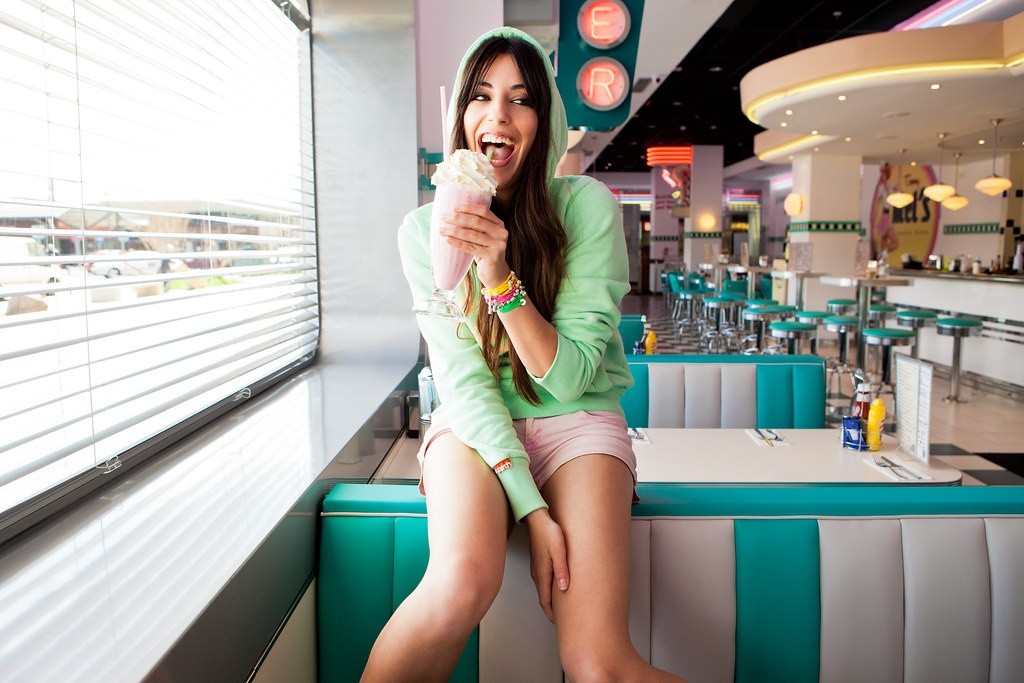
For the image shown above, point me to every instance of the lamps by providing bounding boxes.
[975,118,1013,197]
[783,192,803,215]
[886,148,914,208]
[922,133,957,202]
[940,153,970,211]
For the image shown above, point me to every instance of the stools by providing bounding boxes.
[869,304,898,375]
[698,298,736,357]
[897,311,938,361]
[769,321,818,355]
[745,297,779,347]
[795,311,835,355]
[769,304,796,348]
[936,318,983,404]
[825,298,859,367]
[822,315,861,396]
[863,328,915,432]
[742,308,780,356]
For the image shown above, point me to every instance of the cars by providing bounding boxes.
[85,249,160,280]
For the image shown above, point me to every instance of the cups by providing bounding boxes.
[412,183,493,323]
[418,373,441,446]
[865,268,877,279]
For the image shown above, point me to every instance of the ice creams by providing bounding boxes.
[429,149,498,289]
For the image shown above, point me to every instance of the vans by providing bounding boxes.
[0,236,72,300]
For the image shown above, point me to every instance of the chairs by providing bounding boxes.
[659,266,773,343]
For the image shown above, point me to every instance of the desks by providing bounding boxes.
[772,268,825,355]
[820,273,916,392]
[730,266,771,343]
[369,430,965,487]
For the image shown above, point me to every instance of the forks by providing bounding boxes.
[872,454,907,480]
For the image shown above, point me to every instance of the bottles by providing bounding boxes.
[1012,240,1023,273]
[854,383,871,420]
[646,331,658,355]
[642,321,653,346]
[868,398,887,451]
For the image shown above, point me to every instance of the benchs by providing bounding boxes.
[617,355,826,428]
[317,482,1024,683]
[617,314,646,356]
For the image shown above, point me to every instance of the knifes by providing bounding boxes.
[881,456,922,479]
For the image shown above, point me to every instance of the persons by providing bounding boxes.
[358,26,683,683]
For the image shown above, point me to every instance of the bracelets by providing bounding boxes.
[481,269,527,314]
